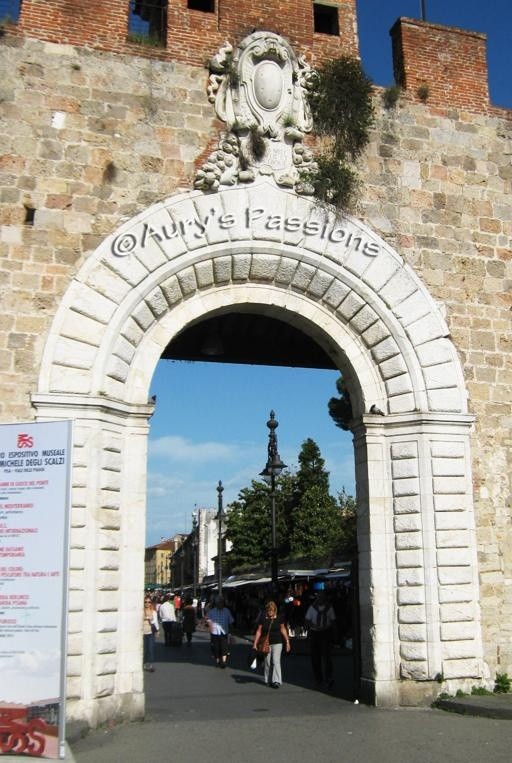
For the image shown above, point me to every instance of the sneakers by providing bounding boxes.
[216,662,228,668]
[266,682,280,688]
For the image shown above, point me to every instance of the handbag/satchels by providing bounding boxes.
[258,635,270,653]
[151,624,157,633]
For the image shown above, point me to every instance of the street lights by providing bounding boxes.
[259,409,288,597]
[213,479,228,594]
[158,551,175,592]
[180,536,185,595]
[192,504,200,597]
[160,561,164,588]
[165,555,169,587]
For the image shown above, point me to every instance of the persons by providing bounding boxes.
[141,581,356,688]
